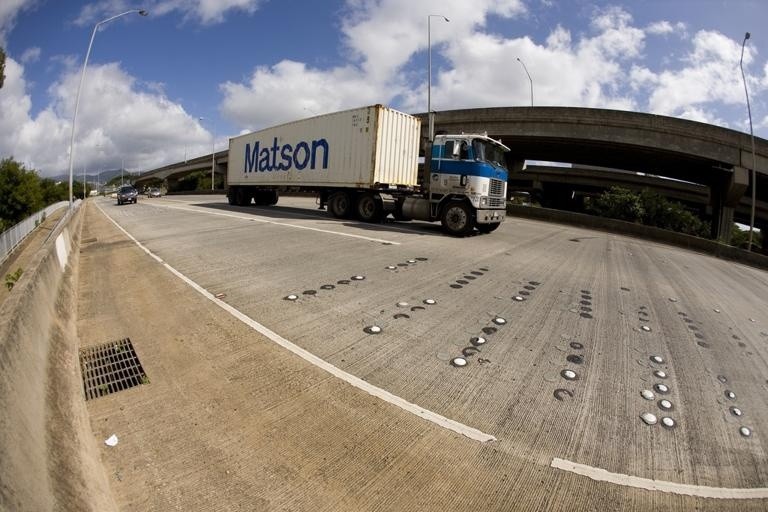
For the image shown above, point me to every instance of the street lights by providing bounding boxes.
[737,29,758,256]
[427,13,451,114]
[515,56,533,109]
[67,7,150,210]
[198,115,215,192]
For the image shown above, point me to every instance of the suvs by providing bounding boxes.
[87,183,163,206]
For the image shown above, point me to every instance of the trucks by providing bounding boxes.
[224,103,518,239]
